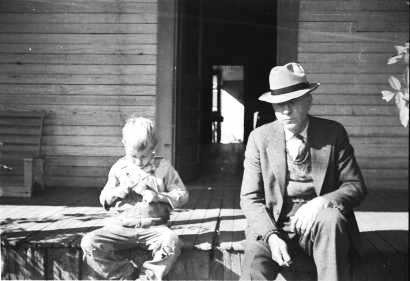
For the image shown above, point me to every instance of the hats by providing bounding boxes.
[258,62,321,104]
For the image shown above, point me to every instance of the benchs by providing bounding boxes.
[0,110,48,199]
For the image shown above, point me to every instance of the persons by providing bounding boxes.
[239,62,367,281]
[81,117,189,281]
